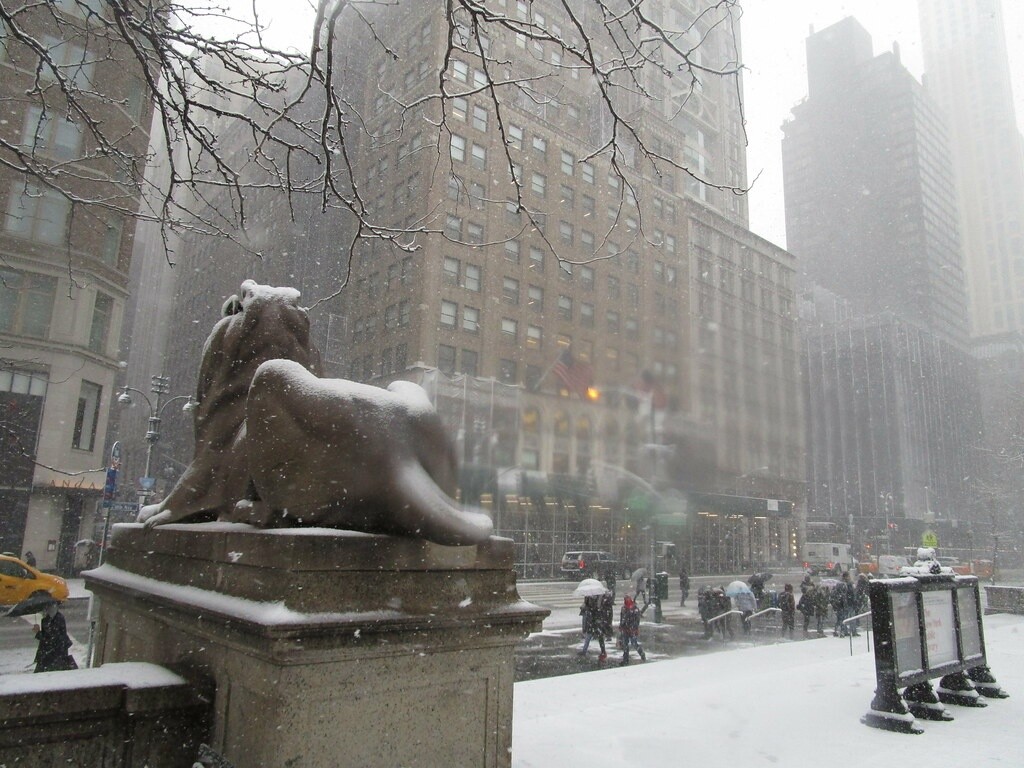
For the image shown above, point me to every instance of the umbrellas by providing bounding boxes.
[74,539,97,547]
[1,595,64,619]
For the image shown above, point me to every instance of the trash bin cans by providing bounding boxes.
[656,573,669,599]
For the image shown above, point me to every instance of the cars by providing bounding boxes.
[0,551,69,608]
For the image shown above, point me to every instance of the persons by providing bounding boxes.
[83,544,98,570]
[32,601,73,674]
[569,560,901,671]
[25,551,36,574]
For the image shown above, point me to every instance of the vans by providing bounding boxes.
[936,556,960,566]
[561,551,618,579]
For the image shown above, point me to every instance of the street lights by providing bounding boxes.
[880,492,892,554]
[117,372,197,513]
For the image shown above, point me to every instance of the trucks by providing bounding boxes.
[802,542,873,576]
[878,555,914,577]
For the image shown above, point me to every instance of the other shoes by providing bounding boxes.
[580,626,861,668]
